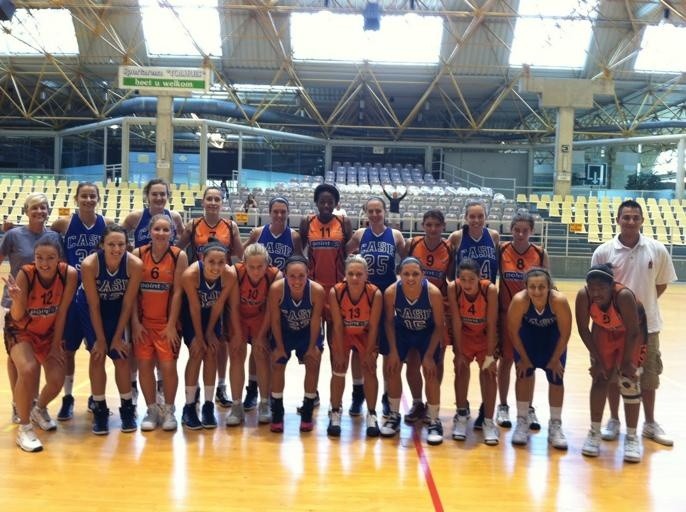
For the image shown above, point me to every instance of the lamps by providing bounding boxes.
[635,2,663,25]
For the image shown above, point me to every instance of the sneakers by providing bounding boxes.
[380,412,401,437]
[13,403,21,424]
[88,396,113,415]
[226,404,245,427]
[119,398,136,432]
[258,401,271,424]
[528,406,540,430]
[624,435,642,463]
[474,405,484,430]
[382,393,389,418]
[582,429,602,457]
[162,407,177,430]
[141,407,159,430]
[301,397,314,431]
[327,408,341,436]
[90,399,109,434]
[181,402,202,430]
[547,420,568,450]
[427,415,443,445]
[296,390,320,415]
[601,419,620,440]
[422,402,441,424]
[481,418,499,445]
[215,387,233,408]
[270,396,284,432]
[512,422,530,445]
[496,405,512,428]
[405,401,424,424]
[328,404,342,416]
[202,401,217,429]
[453,410,468,441]
[16,423,44,452]
[194,387,200,411]
[132,380,138,408]
[147,391,175,415]
[642,422,673,446]
[349,384,363,416]
[30,407,57,432]
[453,402,470,422]
[243,380,258,411]
[57,394,74,420]
[366,410,379,437]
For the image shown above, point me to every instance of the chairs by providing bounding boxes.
[616,225,622,234]
[202,185,207,191]
[666,220,677,227]
[172,204,184,214]
[624,197,633,201]
[600,210,611,218]
[528,194,539,203]
[661,206,672,214]
[411,164,423,186]
[671,239,682,246]
[183,197,195,207]
[0,205,9,215]
[66,201,76,209]
[643,218,652,226]
[602,225,613,235]
[659,199,669,207]
[588,216,599,226]
[602,234,613,244]
[653,218,664,227]
[548,208,560,218]
[46,222,54,227]
[170,191,182,199]
[96,201,104,210]
[358,161,372,185]
[651,212,663,219]
[516,194,527,203]
[169,182,179,192]
[130,183,138,191]
[9,206,22,216]
[1,179,10,187]
[575,223,587,234]
[96,190,105,197]
[674,207,684,213]
[438,178,449,188]
[182,190,193,199]
[560,209,573,219]
[118,183,129,191]
[380,163,392,185]
[559,216,573,226]
[0,186,8,193]
[69,180,79,188]
[541,194,551,204]
[117,219,126,227]
[96,208,103,215]
[107,190,118,197]
[670,229,681,239]
[588,225,599,234]
[21,187,31,193]
[56,181,67,189]
[588,210,598,217]
[677,214,686,220]
[536,201,547,210]
[612,197,622,205]
[47,193,54,200]
[549,200,560,210]
[683,227,686,236]
[573,203,586,210]
[656,225,667,236]
[612,204,620,212]
[55,194,66,201]
[106,209,117,218]
[119,196,131,204]
[368,162,381,185]
[574,210,586,217]
[45,180,56,188]
[170,196,183,205]
[649,205,660,213]
[208,185,546,236]
[120,190,131,196]
[640,206,648,213]
[642,225,654,235]
[657,234,669,245]
[179,184,188,193]
[424,173,436,187]
[671,199,681,207]
[21,214,30,220]
[46,187,56,194]
[6,215,17,226]
[17,192,29,200]
[11,179,22,187]
[106,196,118,203]
[564,196,574,205]
[48,216,58,223]
[681,199,685,207]
[600,204,610,211]
[106,182,118,191]
[0,215,5,225]
[191,183,200,193]
[613,211,619,218]
[587,197,597,204]
[664,214,674,220]
[600,197,610,204]
[132,203,144,211]
[53,200,64,208]
[92,181,105,190]
[670,226,680,236]
[23,179,33,187]
[13,199,26,207]
[51,208,61,217]
[587,203,598,211]
[576,195,587,204]
[69,209,76,215]
[34,187,44,193]
[69,188,78,195]
[132,190,143,196]
[5,192,16,200]
[332,160,352,185]
[642,212,650,219]
[347,160,361,185]
[118,203,131,211]
[646,198,657,206]
[58,188,68,194]
[8,186,20,193]
[679,220,686,228]
[106,203,117,210]
[636,197,646,207]
[401,162,412,186]
[561,203,572,210]
[0,193,5,200]
[2,199,13,207]
[553,194,562,205]
[195,192,204,200]
[132,196,143,204]
[274,171,335,186]
[99,196,105,203]
[118,210,131,219]
[67,195,78,202]
[389,163,402,186]
[587,233,600,243]
[34,179,44,188]
[575,216,586,225]
[19,219,30,226]
[602,217,613,225]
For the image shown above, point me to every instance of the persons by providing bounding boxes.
[448,259,499,446]
[332,202,346,216]
[250,184,353,412]
[507,268,572,450]
[268,251,325,433]
[344,197,405,416]
[576,263,649,463]
[225,243,283,427]
[181,236,237,431]
[591,201,677,445]
[448,202,500,429]
[132,214,188,432]
[175,187,248,408]
[221,178,228,199]
[404,211,455,424]
[0,192,66,425]
[2,182,115,420]
[380,257,445,445]
[76,224,143,435]
[1,235,78,452]
[240,193,257,213]
[381,184,408,230]
[496,213,550,430]
[242,197,308,411]
[118,179,186,417]
[327,253,382,438]
